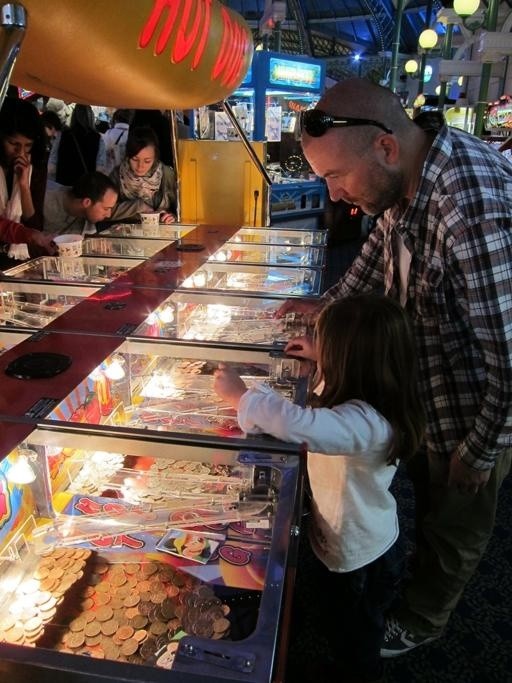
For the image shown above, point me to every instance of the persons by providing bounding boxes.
[274,77,511,657]
[1,96,177,265]
[214,290,429,681]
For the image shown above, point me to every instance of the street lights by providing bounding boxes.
[406,0,500,139]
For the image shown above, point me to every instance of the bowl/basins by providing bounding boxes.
[138,211,161,225]
[52,234,84,257]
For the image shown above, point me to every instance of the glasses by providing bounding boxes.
[299,110,392,137]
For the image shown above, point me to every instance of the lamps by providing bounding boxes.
[404,0,499,139]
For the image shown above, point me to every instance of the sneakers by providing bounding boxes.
[377,609,441,658]
